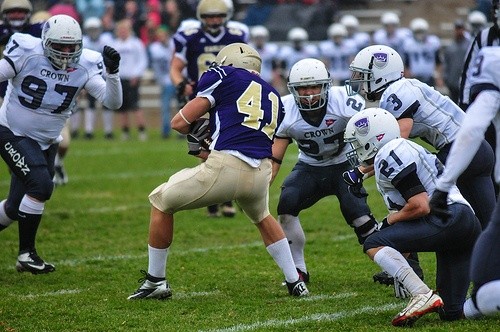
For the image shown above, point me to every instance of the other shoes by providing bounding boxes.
[223,206,236,218]
[72,132,147,143]
[162,134,169,139]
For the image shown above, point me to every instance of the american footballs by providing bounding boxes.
[186,118,212,150]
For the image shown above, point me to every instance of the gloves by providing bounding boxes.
[342,167,365,185]
[428,189,452,224]
[102,46,121,74]
[187,118,212,155]
[349,180,369,199]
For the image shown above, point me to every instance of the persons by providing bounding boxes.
[342,45,496,232]
[341,106,480,325]
[0,0,198,185]
[268,57,379,283]
[128,43,309,298]
[456,0,500,109]
[170,0,249,218]
[428,44,499,309]
[250,10,486,94]
[0,14,123,274]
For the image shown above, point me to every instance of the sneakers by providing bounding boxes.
[127,269,172,299]
[16,251,55,275]
[373,259,424,285]
[393,292,443,327]
[297,267,309,282]
[284,276,308,298]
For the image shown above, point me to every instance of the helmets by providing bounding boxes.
[468,11,486,28]
[251,12,430,50]
[344,107,401,169]
[286,58,332,111]
[198,0,233,29]
[345,45,404,102]
[2,1,31,27]
[41,13,83,71]
[207,42,263,73]
